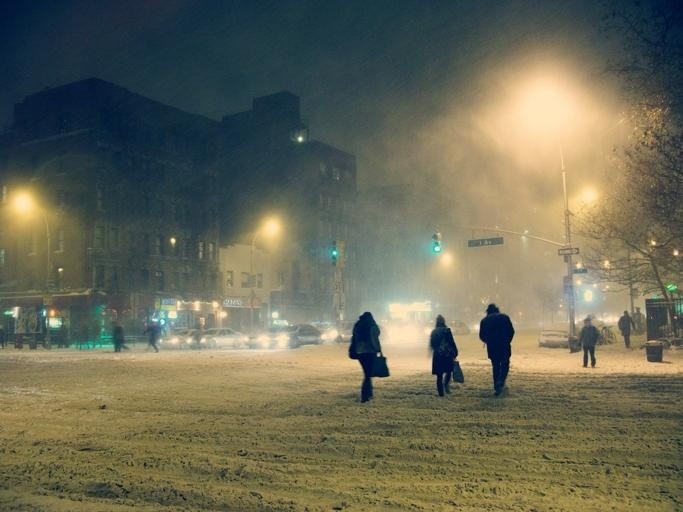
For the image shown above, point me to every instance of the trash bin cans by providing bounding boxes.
[30,339,38,350]
[645,341,664,362]
[15,340,23,348]
[43,338,53,349]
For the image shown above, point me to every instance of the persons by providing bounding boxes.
[617,310,636,348]
[0,324,5,348]
[479,303,514,395]
[578,318,599,368]
[111,321,123,351]
[91,319,102,349]
[428,314,458,397]
[348,312,380,403]
[143,321,164,353]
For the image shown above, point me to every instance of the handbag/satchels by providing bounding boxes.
[370,354,390,378]
[451,361,464,384]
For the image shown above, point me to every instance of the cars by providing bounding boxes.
[158,321,357,349]
[447,320,470,335]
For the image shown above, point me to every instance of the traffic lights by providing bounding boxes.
[432,233,443,254]
[331,240,337,265]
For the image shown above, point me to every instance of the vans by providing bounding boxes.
[538,330,568,348]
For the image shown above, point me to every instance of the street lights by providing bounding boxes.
[251,215,282,332]
[511,81,574,353]
[4,187,50,277]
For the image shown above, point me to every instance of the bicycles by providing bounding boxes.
[601,325,617,345]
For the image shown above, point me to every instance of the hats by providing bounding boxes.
[436,314,445,325]
[485,304,496,312]
